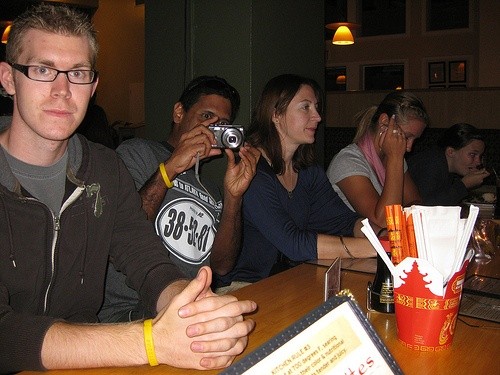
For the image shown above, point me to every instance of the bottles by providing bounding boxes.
[370,235,394,314]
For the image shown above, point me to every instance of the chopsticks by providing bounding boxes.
[361,203,480,282]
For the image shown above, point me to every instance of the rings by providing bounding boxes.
[398,133,401,135]
[393,130,398,133]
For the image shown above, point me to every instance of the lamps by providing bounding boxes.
[335,72,346,84]
[325,17,362,46]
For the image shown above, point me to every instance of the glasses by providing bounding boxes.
[10,62,96,85]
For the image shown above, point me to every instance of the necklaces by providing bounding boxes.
[272,161,293,199]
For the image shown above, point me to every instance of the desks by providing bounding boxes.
[22,207,500,375]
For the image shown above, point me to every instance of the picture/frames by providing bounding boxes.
[428,60,466,84]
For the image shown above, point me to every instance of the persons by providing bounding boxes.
[0,3,257,375]
[214,73,391,294]
[326,91,429,228]
[98,75,261,322]
[406,122,490,206]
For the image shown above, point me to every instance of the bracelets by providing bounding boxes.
[340,235,353,258]
[144,318,159,366]
[378,228,387,239]
[159,163,174,188]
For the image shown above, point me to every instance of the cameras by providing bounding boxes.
[208,124,244,148]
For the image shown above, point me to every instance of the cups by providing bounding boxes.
[467,218,498,265]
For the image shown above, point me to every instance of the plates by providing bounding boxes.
[462,198,495,209]
